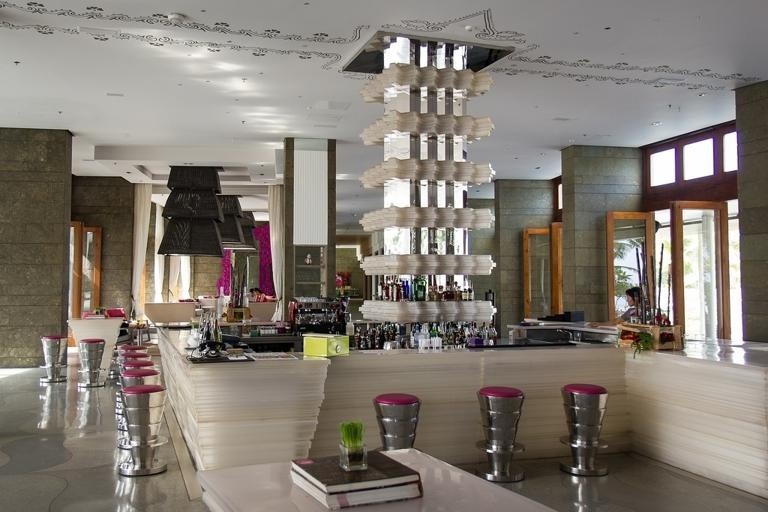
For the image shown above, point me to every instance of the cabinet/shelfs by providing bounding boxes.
[358,63,498,325]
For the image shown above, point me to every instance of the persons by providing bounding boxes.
[588,286,645,328]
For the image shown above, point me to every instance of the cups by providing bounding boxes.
[230,319,252,337]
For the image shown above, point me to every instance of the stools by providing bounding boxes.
[372,383,613,485]
[35,334,169,477]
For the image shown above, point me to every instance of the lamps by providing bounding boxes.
[156,166,256,258]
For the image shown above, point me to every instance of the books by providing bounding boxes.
[289,449,425,509]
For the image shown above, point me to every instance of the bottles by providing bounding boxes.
[203,318,222,345]
[346,320,497,349]
[377,275,473,300]
[233,288,244,308]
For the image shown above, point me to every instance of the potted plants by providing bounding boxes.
[338,418,368,473]
